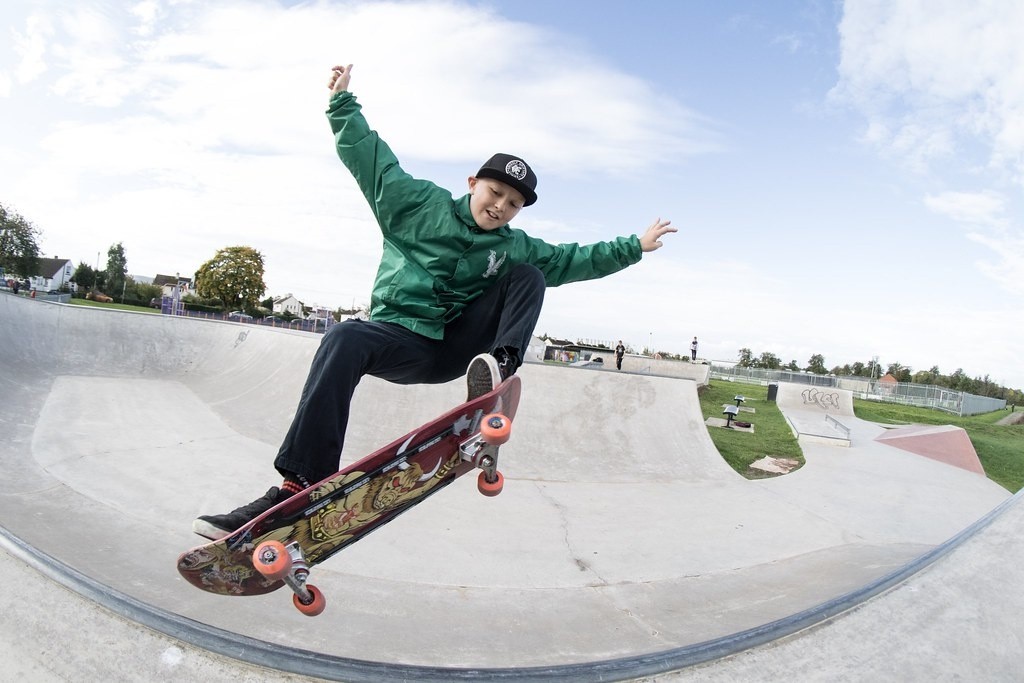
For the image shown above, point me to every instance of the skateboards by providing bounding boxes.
[175,374,522,620]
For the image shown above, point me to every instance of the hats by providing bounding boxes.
[475,153,538,207]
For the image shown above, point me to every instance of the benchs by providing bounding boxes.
[721,405,739,429]
[734,395,744,408]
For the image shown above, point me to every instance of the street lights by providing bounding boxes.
[95,252,101,288]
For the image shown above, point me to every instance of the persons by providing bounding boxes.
[189,63,677,541]
[615,340,626,371]
[689,336,699,364]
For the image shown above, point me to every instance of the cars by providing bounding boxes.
[229,310,253,321]
[149,297,165,309]
[291,318,309,326]
[93,290,113,302]
[265,315,286,325]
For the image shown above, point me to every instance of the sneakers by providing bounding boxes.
[466,353,504,402]
[193,486,284,541]
